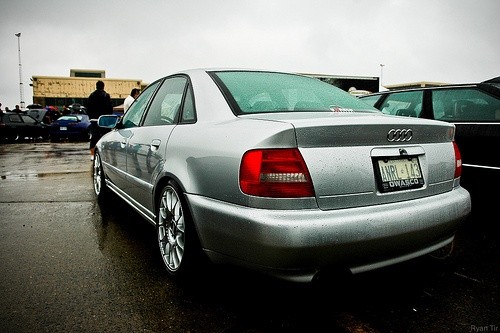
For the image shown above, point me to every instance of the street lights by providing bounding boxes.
[15,32,23,108]
[379,64,385,88]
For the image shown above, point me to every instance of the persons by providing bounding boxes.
[124,88,140,114]
[11,105,22,112]
[5,107,11,112]
[42,104,86,125]
[86,81,113,161]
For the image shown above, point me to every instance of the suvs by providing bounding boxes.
[358,83,500,208]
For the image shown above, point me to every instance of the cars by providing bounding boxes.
[50,114,92,142]
[92,66,472,280]
[0,109,50,143]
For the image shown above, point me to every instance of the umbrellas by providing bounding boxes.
[26,104,42,109]
[68,103,85,109]
[44,106,58,111]
